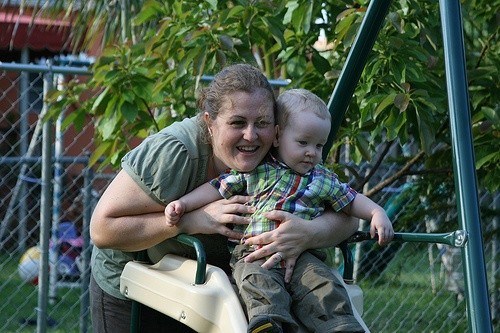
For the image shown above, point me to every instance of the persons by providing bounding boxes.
[87,64,279,333]
[164,88,395,333]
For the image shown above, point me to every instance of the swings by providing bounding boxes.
[0,0,493,333]
